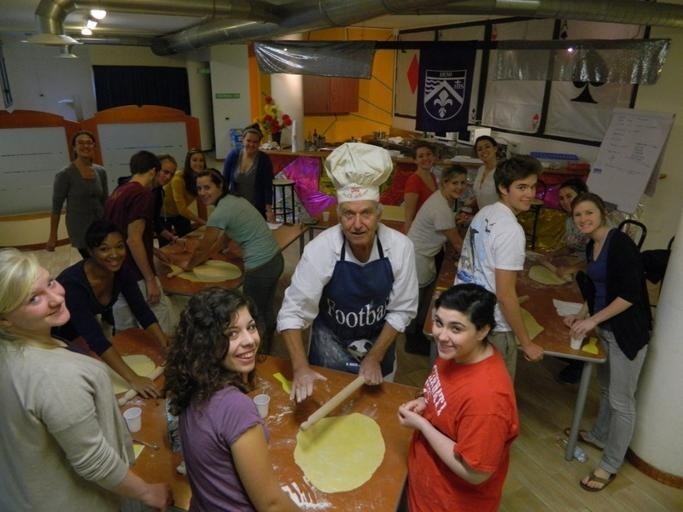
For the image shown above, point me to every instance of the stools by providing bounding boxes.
[272,178,297,225]
[519,198,545,250]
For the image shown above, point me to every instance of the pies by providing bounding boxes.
[515,305,545,345]
[529,265,571,285]
[106,355,155,395]
[293,413,386,494]
[169,258,242,282]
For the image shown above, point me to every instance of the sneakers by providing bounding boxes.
[558,365,581,384]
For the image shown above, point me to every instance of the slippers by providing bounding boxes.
[580,467,616,492]
[564,427,604,451]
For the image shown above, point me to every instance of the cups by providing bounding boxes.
[122,407,141,432]
[253,394,270,420]
[568,330,584,351]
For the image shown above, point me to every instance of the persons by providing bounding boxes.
[1,248,175,511]
[47,132,108,260]
[163,285,301,510]
[102,124,284,355]
[50,220,176,398]
[276,142,420,403]
[559,178,618,260]
[453,155,545,387]
[397,283,520,511]
[563,192,651,492]
[404,142,445,281]
[404,164,469,357]
[473,135,505,209]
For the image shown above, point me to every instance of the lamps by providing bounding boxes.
[79,8,109,38]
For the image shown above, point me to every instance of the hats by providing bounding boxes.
[323,143,394,204]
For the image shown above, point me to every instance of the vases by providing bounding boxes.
[270,130,281,149]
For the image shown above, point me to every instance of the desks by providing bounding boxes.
[151,217,320,298]
[64,324,430,510]
[421,241,610,468]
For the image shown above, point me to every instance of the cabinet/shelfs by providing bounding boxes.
[302,74,360,116]
[616,219,677,310]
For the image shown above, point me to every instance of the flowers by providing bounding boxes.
[254,90,294,133]
